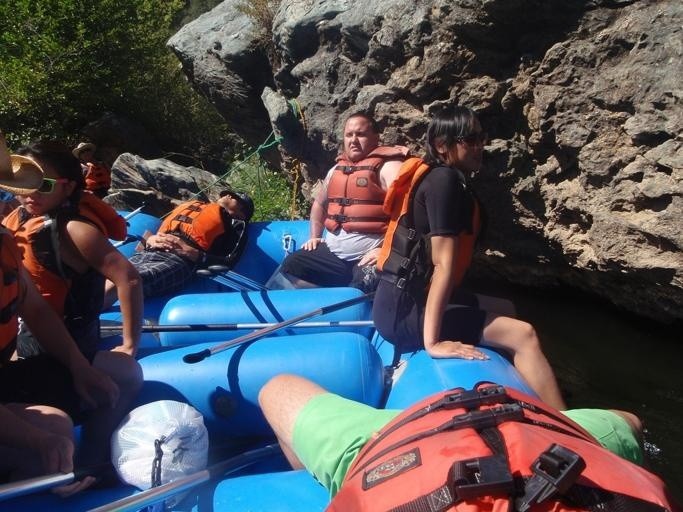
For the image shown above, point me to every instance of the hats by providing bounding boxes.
[0,128,44,196]
[71,142,96,159]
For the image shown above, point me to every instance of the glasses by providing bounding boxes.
[451,132,489,151]
[34,177,68,195]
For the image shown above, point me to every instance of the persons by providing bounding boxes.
[100,189,252,315]
[0,139,143,356]
[370,105,568,413]
[256,372,676,512]
[1,129,145,498]
[71,141,112,197]
[278,113,412,291]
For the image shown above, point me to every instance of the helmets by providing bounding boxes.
[219,189,254,220]
